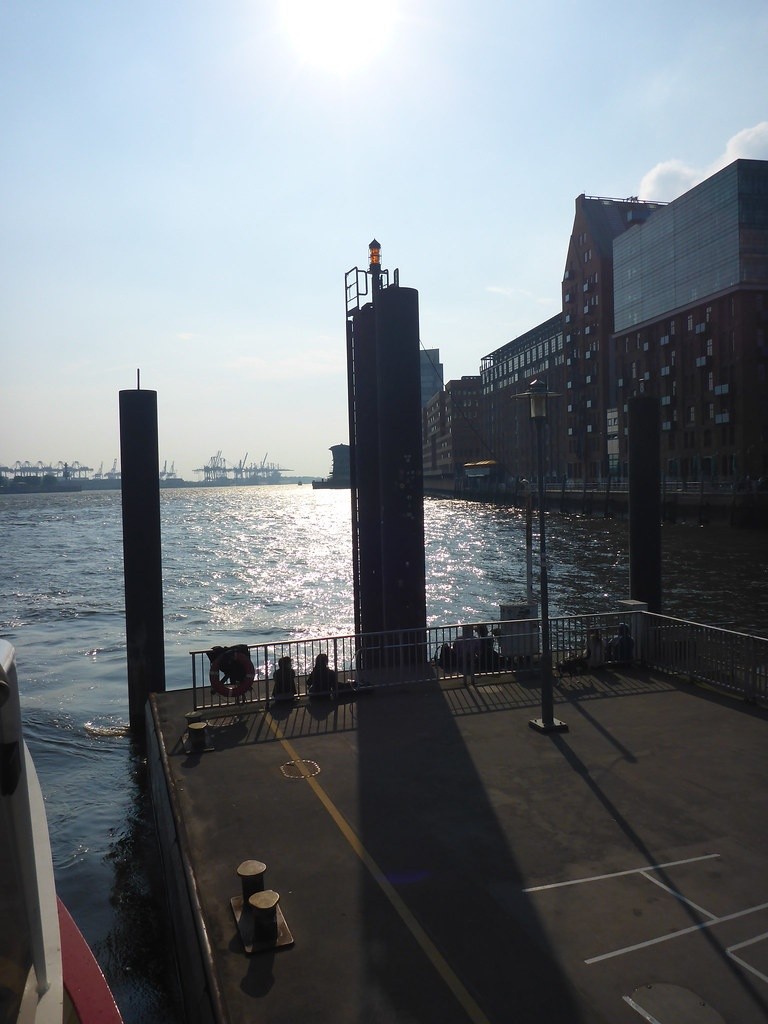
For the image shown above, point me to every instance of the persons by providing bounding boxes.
[454,625,481,672]
[609,623,635,667]
[272,656,297,703]
[475,624,498,671]
[584,629,609,669]
[307,653,337,701]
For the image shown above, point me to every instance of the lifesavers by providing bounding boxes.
[209,648,256,698]
